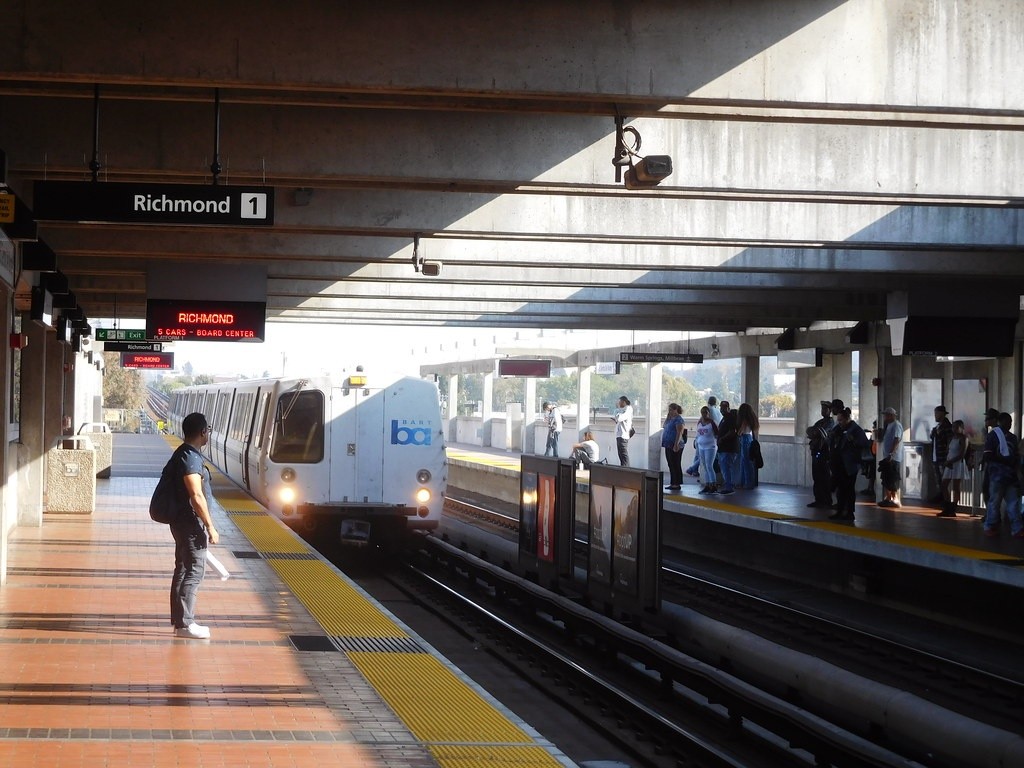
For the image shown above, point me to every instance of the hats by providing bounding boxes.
[879,407,898,416]
[619,396,630,405]
[984,407,999,418]
[935,406,949,414]
[820,401,833,412]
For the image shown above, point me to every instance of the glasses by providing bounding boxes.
[201,426,212,432]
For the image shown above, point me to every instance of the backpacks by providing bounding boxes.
[149,448,204,524]
[959,434,976,470]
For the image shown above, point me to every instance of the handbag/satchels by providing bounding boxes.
[749,440,763,468]
[821,471,839,492]
[560,414,566,426]
[682,428,687,443]
[630,428,635,438]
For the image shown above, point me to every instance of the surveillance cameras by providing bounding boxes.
[422,261,443,276]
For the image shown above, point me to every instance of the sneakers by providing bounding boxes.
[173,622,210,639]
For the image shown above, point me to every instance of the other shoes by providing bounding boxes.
[828,512,855,523]
[936,502,957,517]
[807,500,832,508]
[664,467,735,496]
[1016,528,1024,538]
[984,527,1000,536]
[860,488,875,496]
[879,497,899,507]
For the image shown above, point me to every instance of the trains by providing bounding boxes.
[166,366,449,540]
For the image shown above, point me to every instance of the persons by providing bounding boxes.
[687,396,759,496]
[543,402,562,458]
[166,412,220,639]
[614,396,633,467]
[662,403,685,490]
[806,399,1024,538]
[572,431,599,470]
